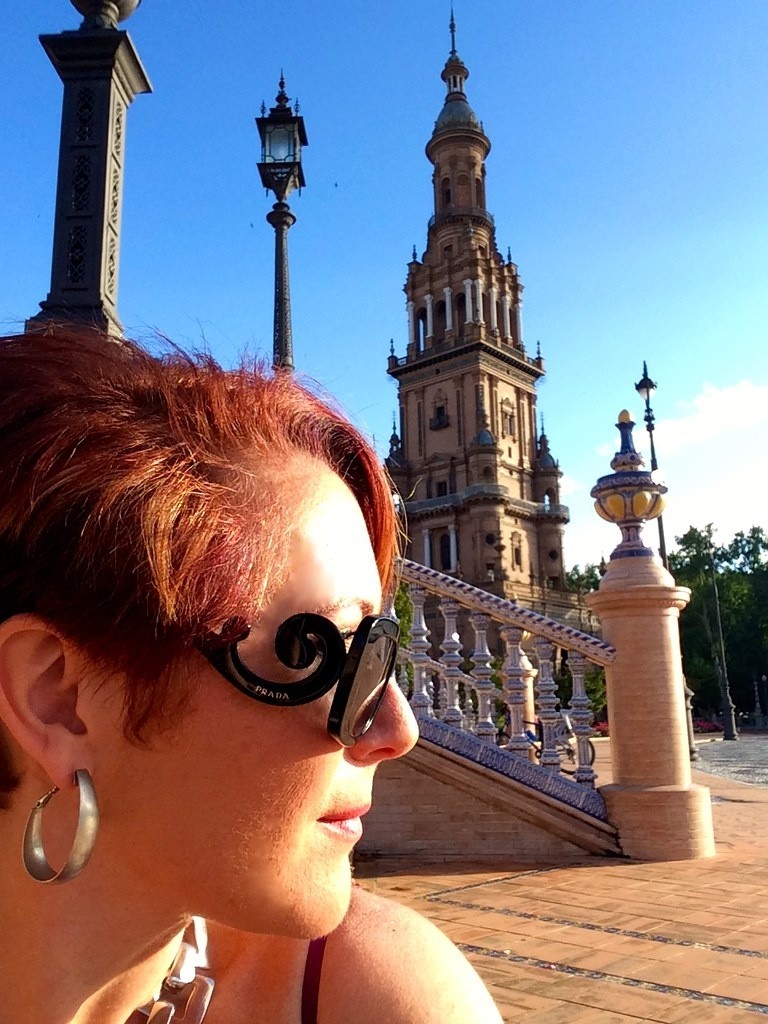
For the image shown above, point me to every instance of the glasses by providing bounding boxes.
[192,612,403,747]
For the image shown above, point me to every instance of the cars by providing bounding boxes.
[693,718,725,732]
[599,705,609,737]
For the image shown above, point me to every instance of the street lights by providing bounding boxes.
[254,65,307,381]
[753,675,767,728]
[634,360,669,571]
[705,558,738,743]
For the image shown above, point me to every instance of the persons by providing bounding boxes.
[0,323,504,1024]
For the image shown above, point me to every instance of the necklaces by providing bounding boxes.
[138,915,216,1024]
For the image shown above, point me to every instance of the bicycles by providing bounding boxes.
[495,703,596,775]
